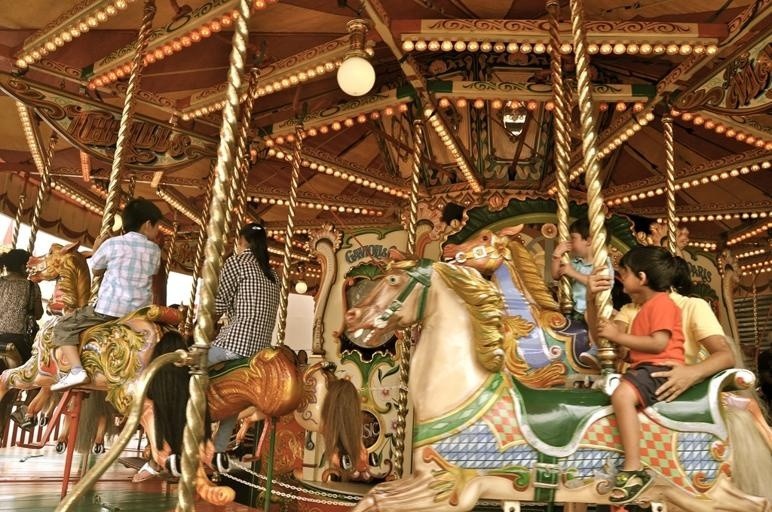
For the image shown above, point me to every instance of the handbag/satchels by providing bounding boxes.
[24,308,39,348]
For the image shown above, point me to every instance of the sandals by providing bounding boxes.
[131,460,166,483]
[607,465,654,504]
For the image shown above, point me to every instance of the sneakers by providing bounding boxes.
[8,405,39,432]
[577,341,607,368]
[49,368,93,393]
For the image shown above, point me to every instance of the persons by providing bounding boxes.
[552,219,615,370]
[0,250,43,433]
[131,223,279,482]
[614,260,736,403]
[587,245,685,504]
[51,199,160,392]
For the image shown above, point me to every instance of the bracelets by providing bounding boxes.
[552,254,561,259]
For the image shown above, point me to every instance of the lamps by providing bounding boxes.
[296,266,307,294]
[337,18,376,97]
